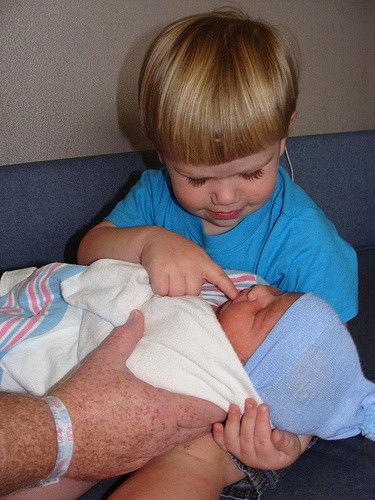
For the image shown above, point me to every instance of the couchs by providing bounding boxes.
[0,128,375,499]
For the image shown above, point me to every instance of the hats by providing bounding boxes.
[244,293,375,442]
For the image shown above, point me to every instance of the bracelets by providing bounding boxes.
[40,395,74,486]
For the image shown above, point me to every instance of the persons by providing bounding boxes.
[76,6,359,500]
[0,257,374,442]
[0,310,229,492]
